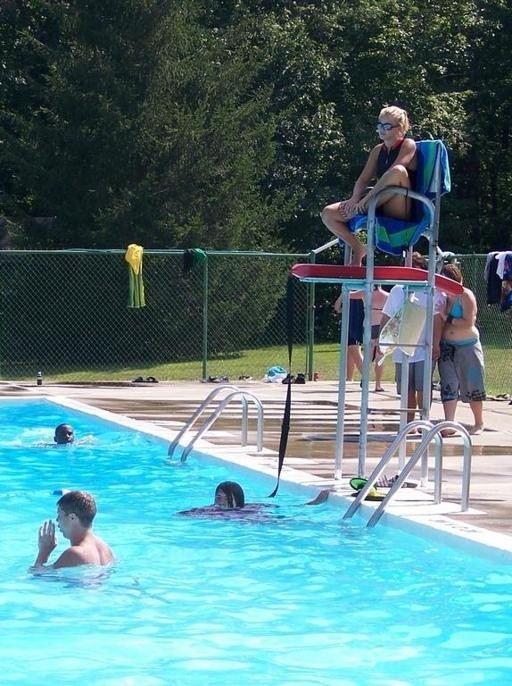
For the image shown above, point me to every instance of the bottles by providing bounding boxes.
[37,371,43,385]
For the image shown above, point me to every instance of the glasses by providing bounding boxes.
[376,123,400,130]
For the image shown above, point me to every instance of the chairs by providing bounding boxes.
[338,138,449,265]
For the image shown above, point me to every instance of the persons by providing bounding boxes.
[33,491,114,567]
[182,480,245,521]
[336,291,367,381]
[334,283,391,391]
[53,424,76,445]
[437,264,487,437]
[321,105,419,266]
[375,251,443,428]
